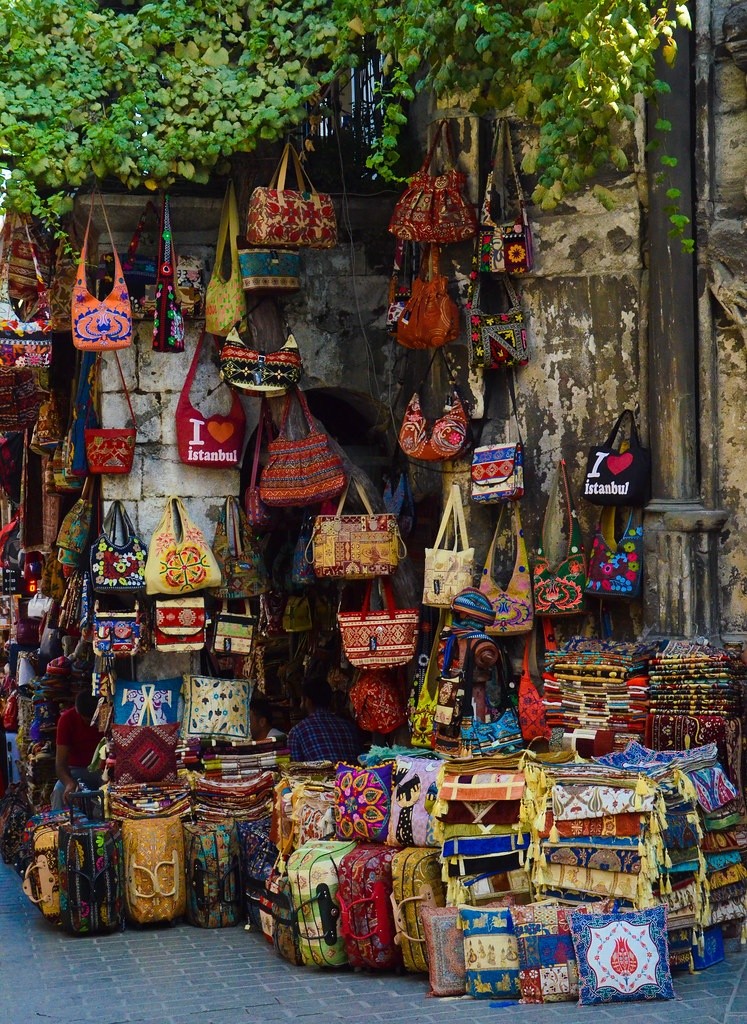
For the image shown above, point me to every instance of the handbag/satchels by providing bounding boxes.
[0,122,747,1004]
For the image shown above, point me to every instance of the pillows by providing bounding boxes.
[420,897,676,1008]
[383,753,443,848]
[333,758,393,843]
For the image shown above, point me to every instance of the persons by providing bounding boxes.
[49,691,103,811]
[286,678,363,766]
[250,701,289,741]
